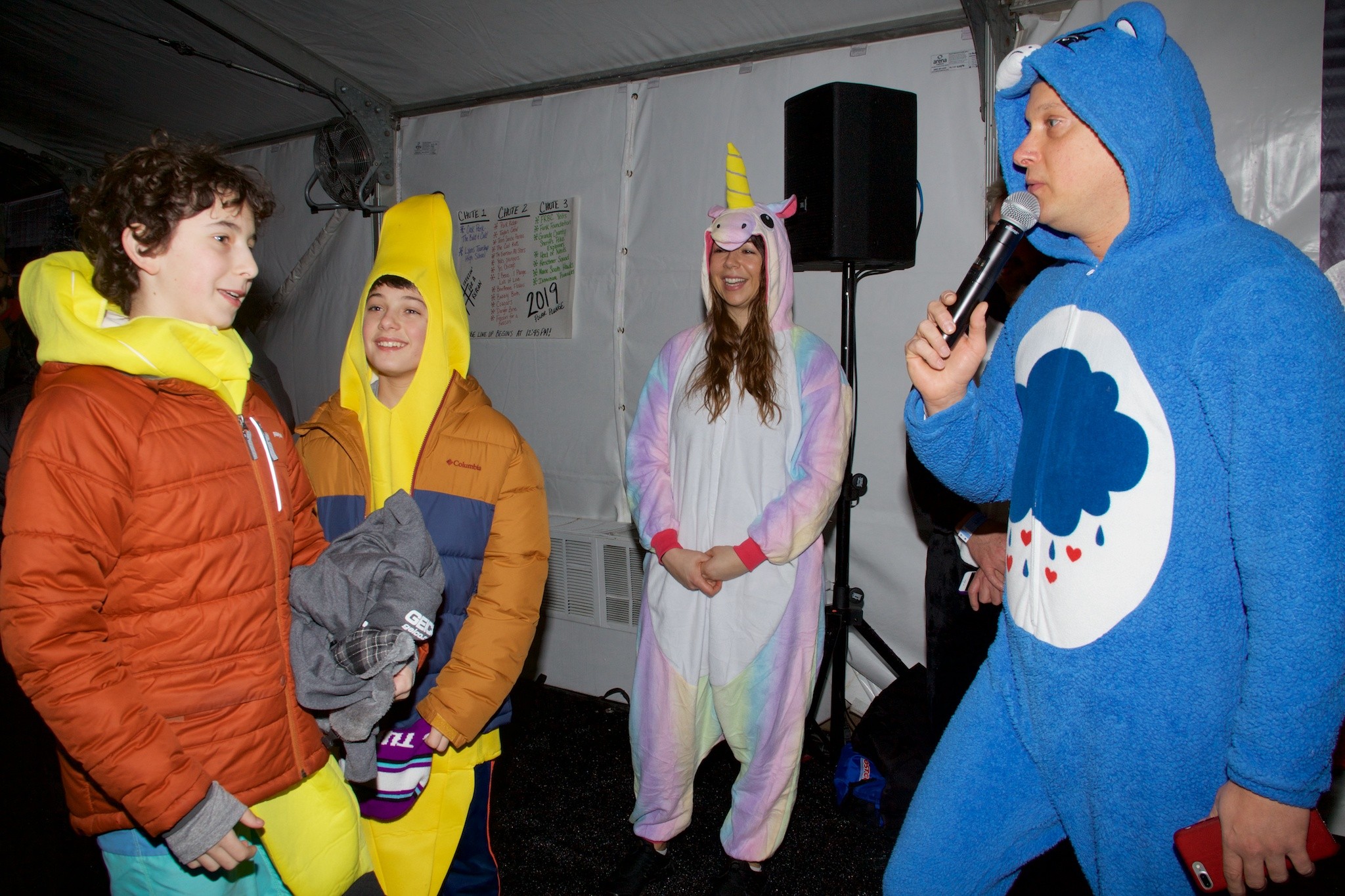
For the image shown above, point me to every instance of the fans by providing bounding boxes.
[304,117,389,217]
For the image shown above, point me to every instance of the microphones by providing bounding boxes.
[935,191,1042,347]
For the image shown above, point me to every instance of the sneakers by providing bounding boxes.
[599,834,674,896]
[706,859,772,896]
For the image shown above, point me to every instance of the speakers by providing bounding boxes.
[782,81,918,272]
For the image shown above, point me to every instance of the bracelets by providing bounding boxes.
[957,513,988,544]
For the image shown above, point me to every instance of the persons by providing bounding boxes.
[603,205,852,896]
[0,134,429,896]
[879,0,1345,896]
[291,271,550,896]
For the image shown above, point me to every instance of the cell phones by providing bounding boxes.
[958,571,979,596]
[1173,804,1340,894]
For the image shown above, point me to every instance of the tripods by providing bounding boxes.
[801,267,909,759]
